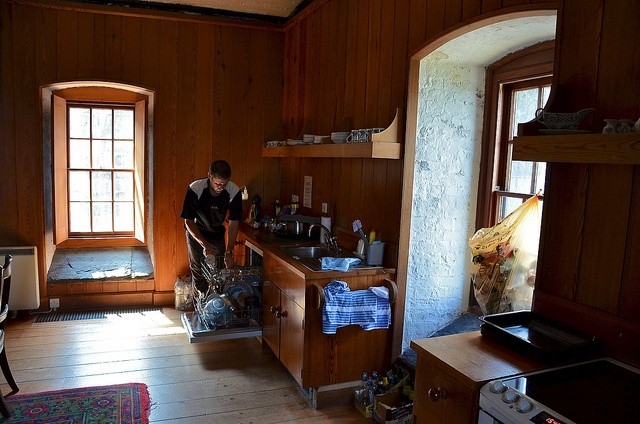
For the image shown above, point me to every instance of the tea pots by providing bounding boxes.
[536,108,594,129]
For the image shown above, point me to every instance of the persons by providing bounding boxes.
[179,160,243,311]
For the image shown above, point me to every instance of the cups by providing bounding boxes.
[365,129,373,142]
[373,128,385,134]
[617,119,633,133]
[602,119,616,133]
[358,129,365,143]
[346,130,358,144]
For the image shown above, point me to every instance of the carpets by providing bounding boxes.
[48,246,155,282]
[0,383,152,424]
[396,313,486,378]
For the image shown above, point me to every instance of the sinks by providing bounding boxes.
[280,246,345,260]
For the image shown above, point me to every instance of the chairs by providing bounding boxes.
[0,254,20,418]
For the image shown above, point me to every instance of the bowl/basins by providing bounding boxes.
[302,133,315,142]
[314,135,330,143]
[203,293,234,326]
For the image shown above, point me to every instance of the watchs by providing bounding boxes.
[224,248,233,256]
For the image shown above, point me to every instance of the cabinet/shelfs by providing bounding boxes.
[262,107,404,160]
[511,113,640,164]
[412,355,476,424]
[224,220,245,266]
[259,251,305,389]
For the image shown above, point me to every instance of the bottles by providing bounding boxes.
[377,375,386,395]
[185,275,193,309]
[173,275,185,310]
[273,198,281,217]
[368,370,377,402]
[359,371,368,404]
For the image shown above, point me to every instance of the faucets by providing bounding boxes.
[307,224,342,255]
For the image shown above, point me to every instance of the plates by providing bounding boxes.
[224,280,254,300]
[331,131,351,144]
[538,130,590,134]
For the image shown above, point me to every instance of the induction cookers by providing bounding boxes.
[477,358,636,424]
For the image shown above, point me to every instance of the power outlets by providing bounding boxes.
[241,192,248,200]
[50,298,60,309]
[321,203,327,212]
[292,195,299,202]
